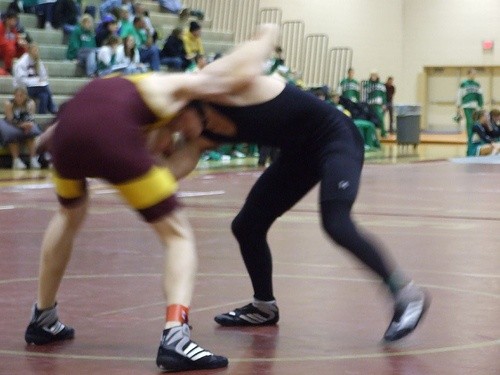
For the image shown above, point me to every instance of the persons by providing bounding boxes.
[0,0,212,117]
[149,23,432,342]
[338,67,361,104]
[456,68,484,149]
[5,85,41,169]
[466,109,500,157]
[184,46,396,161]
[25,68,260,371]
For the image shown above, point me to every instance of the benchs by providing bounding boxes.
[0,0,238,169]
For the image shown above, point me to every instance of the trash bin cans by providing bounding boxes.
[395,104,422,146]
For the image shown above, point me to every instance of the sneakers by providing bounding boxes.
[25,301,75,345]
[214,300,279,326]
[156,324,228,370]
[384,281,430,342]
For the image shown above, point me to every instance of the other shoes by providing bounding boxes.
[12,160,26,169]
[30,155,40,169]
